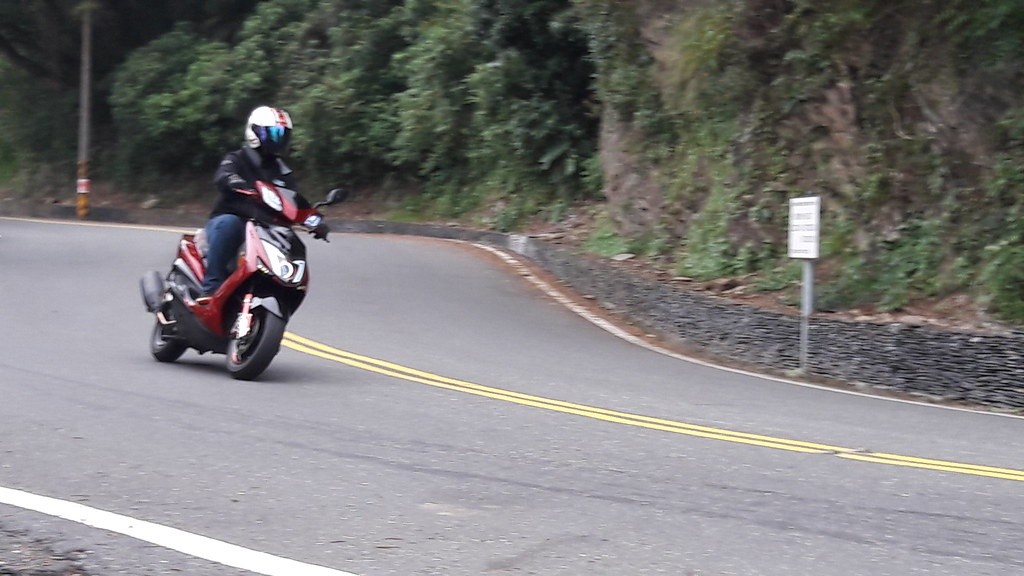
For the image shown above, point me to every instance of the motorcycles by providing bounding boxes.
[139,181,350,381]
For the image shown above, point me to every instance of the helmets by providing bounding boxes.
[245,106,293,156]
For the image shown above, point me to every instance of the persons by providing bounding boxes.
[196,106,330,304]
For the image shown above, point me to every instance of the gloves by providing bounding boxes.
[227,174,247,191]
[308,216,329,239]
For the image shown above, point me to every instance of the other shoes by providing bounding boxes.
[196,291,212,306]
